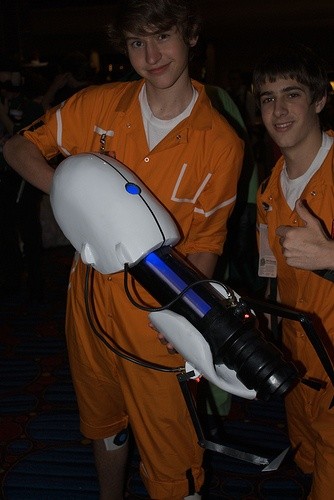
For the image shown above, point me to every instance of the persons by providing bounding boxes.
[2,1,246,500]
[0,4,300,253]
[255,47,334,498]
